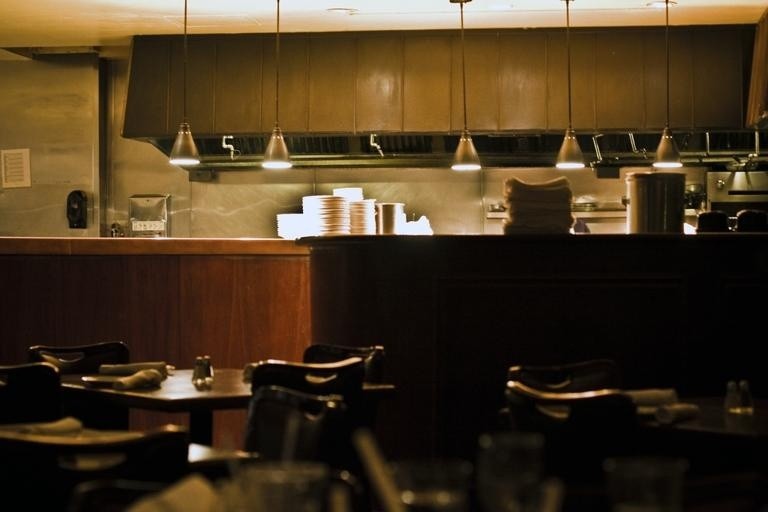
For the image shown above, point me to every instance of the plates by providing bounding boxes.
[333,188,364,203]
[275,213,306,241]
[301,195,350,237]
[80,375,122,384]
[349,198,377,236]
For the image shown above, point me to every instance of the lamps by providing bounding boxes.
[652,0,683,168]
[450,1,482,170]
[555,1,585,169]
[168,1,201,164]
[262,1,293,168]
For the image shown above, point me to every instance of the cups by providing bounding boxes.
[376,201,405,235]
[723,380,754,414]
[243,459,327,512]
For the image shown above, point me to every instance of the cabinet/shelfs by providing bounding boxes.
[1,235,767,451]
[119,34,758,173]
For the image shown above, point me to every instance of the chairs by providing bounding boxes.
[20,339,133,439]
[2,364,202,511]
[302,343,385,383]
[496,382,700,512]
[236,356,371,509]
